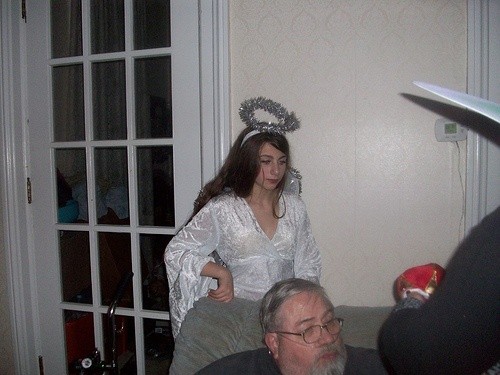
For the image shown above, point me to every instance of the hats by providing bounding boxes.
[393,262,444,300]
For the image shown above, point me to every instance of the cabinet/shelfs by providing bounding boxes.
[60,207,125,306]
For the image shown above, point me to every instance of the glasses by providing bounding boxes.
[273,318,344,345]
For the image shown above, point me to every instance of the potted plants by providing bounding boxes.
[57,168,79,223]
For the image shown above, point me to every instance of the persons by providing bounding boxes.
[377,205,500,375]
[163,122,320,340]
[192,278,387,374]
